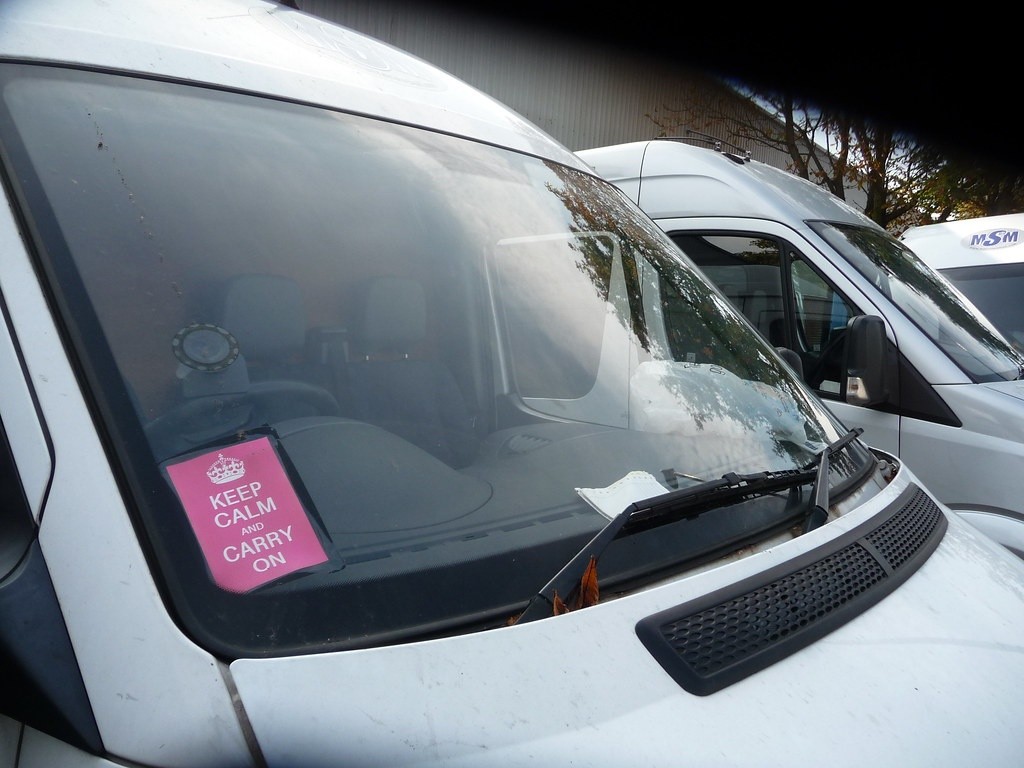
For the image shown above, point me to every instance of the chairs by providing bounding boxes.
[163,273,350,437]
[340,273,482,477]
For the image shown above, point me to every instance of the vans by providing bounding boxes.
[894,213,1024,355]
[0,0,1022,767]
[493,129,1023,561]
